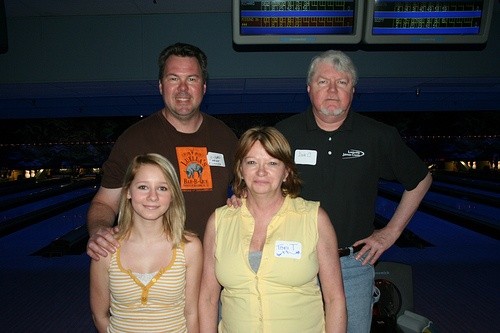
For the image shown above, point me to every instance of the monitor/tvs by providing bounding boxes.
[362,0,495,44]
[232,0,365,44]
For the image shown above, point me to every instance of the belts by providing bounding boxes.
[338,242,370,258]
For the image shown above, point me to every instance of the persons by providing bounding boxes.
[90,153,204,333]
[86,42,238,261]
[226,50,432,333]
[199,126,348,333]
